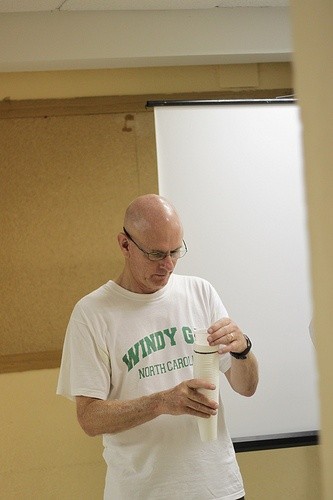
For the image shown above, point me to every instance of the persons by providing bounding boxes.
[51,192,261,500]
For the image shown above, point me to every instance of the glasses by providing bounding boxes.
[124,227,187,262]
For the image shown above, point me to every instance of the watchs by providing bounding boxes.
[224,334,253,360]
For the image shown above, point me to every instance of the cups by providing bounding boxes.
[191,328,220,444]
